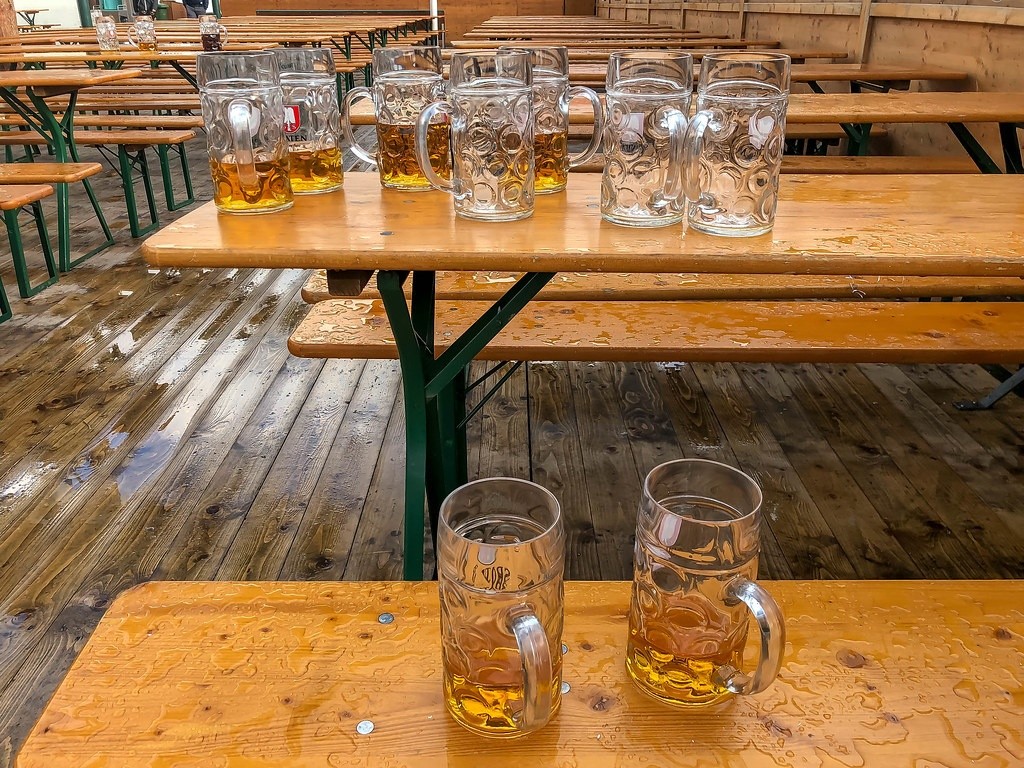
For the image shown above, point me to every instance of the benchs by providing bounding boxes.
[301,269,1024,302]
[288,299,1023,580]
[569,149,979,175]
[18,27,32,33]
[568,125,887,156]
[0,30,450,323]
[18,24,61,29]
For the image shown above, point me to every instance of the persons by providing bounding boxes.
[133,0,158,21]
[182,0,209,18]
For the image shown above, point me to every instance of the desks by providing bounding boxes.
[471,28,698,49]
[462,33,729,49]
[0,36,56,47]
[449,38,780,49]
[351,91,1024,176]
[482,15,643,24]
[18,16,447,113]
[437,46,846,65]
[0,51,200,92]
[440,61,966,156]
[474,25,673,49]
[472,23,659,49]
[11,579,1024,768]
[0,43,279,55]
[0,69,143,275]
[140,172,1024,580]
[15,8,49,30]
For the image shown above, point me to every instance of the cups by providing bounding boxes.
[681,53,791,236]
[342,47,448,192]
[626,458,786,708]
[602,50,694,227]
[132,16,155,40]
[197,51,294,216]
[434,477,568,737]
[199,14,228,51]
[129,22,157,51]
[415,53,536,222]
[496,47,602,195]
[95,15,120,51]
[259,48,344,195]
